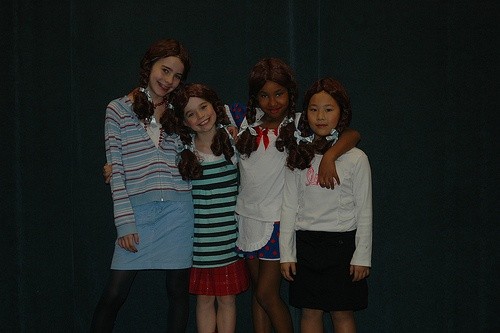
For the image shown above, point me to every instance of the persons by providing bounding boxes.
[222,60,363,333]
[279,77,374,333]
[103,84,249,333]
[89,40,238,333]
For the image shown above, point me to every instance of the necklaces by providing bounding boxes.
[143,94,168,150]
